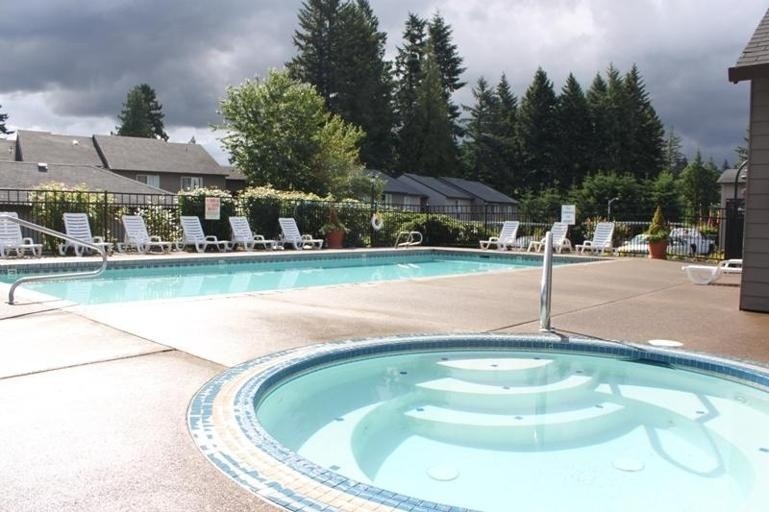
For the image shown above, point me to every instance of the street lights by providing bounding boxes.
[368,175,376,247]
[607,197,620,221]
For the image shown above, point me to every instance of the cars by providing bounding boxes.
[618,226,716,260]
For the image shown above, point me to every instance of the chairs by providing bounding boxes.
[478,219,614,257]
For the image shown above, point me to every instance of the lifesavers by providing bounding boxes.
[372,215,384,229]
